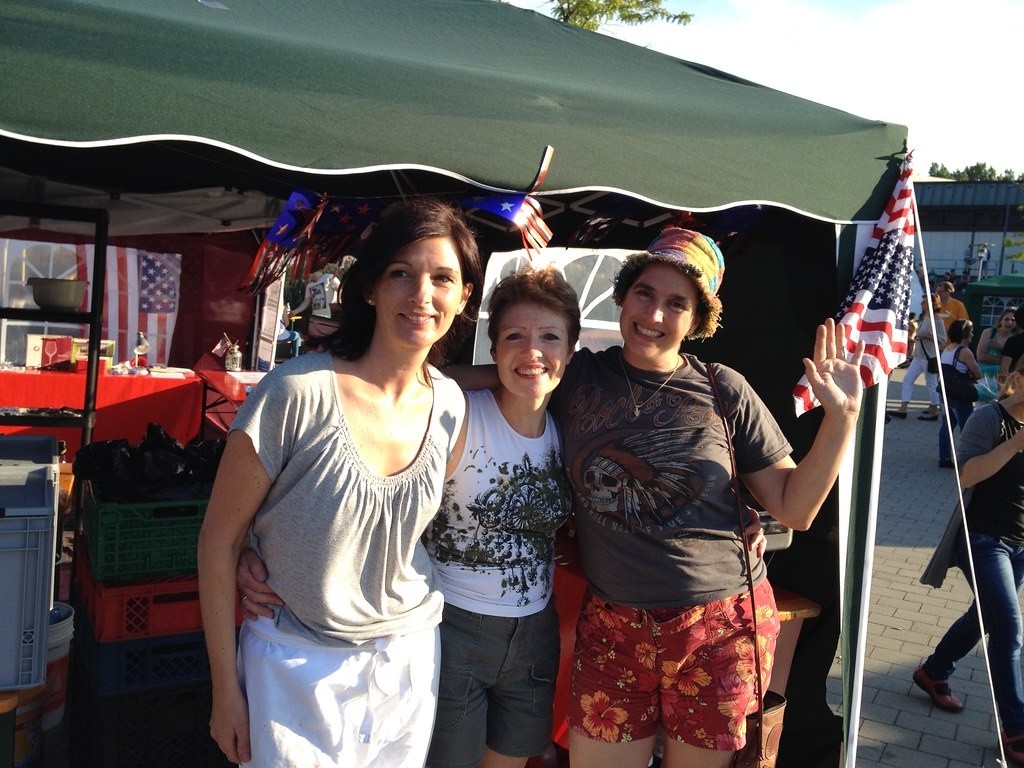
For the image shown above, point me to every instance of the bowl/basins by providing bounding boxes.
[28,277,88,311]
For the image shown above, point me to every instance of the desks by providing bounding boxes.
[0,369,206,465]
[288,316,303,355]
[196,370,271,442]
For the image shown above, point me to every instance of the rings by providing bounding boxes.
[240,593,247,600]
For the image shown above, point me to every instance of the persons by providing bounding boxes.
[918,263,970,294]
[235,265,768,768]
[976,307,1018,408]
[912,352,1024,768]
[437,228,865,768]
[195,202,482,768]
[998,301,1024,383]
[276,304,302,358]
[964,244,977,265]
[981,243,990,278]
[289,263,343,318]
[936,318,981,467]
[885,280,970,424]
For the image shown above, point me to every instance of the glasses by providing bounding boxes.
[935,290,946,294]
[1003,317,1016,322]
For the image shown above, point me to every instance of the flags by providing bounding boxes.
[75,244,180,365]
[791,155,915,417]
[211,337,231,357]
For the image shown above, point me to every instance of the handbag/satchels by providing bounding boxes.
[938,346,980,405]
[928,356,939,374]
[735,689,787,768]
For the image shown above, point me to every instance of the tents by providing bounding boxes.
[966,273,1024,353]
[0,0,908,768]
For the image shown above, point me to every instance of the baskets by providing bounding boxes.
[71,475,234,768]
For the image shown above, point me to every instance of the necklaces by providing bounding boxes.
[619,351,680,417]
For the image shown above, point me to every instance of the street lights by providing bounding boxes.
[970,243,996,282]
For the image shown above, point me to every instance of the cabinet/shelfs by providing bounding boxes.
[0,200,113,465]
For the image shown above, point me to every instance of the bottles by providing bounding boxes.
[225,345,242,372]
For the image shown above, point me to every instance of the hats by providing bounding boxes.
[648,228,725,296]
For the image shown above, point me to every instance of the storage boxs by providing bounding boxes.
[74,469,243,768]
[0,433,60,694]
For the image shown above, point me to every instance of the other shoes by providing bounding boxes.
[997,732,1024,768]
[887,411,907,419]
[922,410,930,414]
[917,414,938,421]
[913,667,963,714]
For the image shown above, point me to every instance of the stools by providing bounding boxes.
[275,341,292,363]
